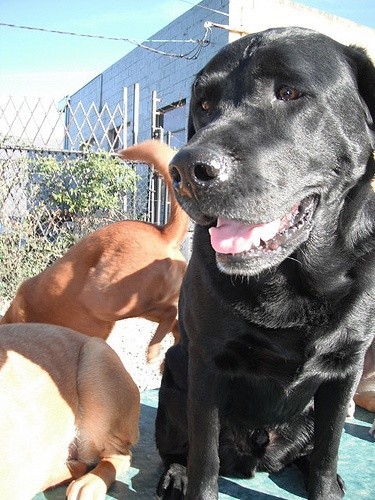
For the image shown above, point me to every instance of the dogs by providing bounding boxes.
[0,323,139,499]
[0,138,190,391]
[154,27,375,500]
